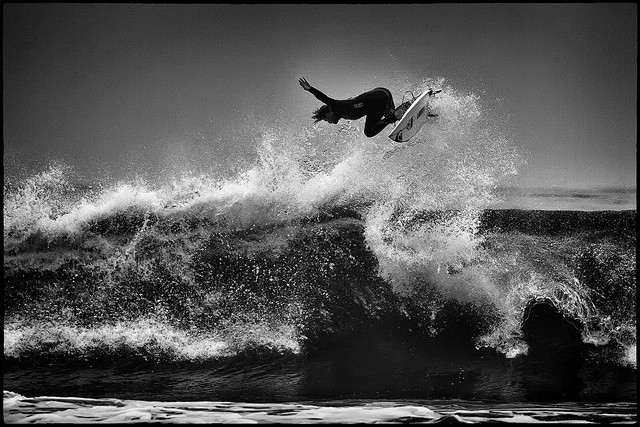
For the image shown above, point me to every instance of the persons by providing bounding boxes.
[297,76,411,138]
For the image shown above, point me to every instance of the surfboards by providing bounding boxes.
[389,87,442,142]
